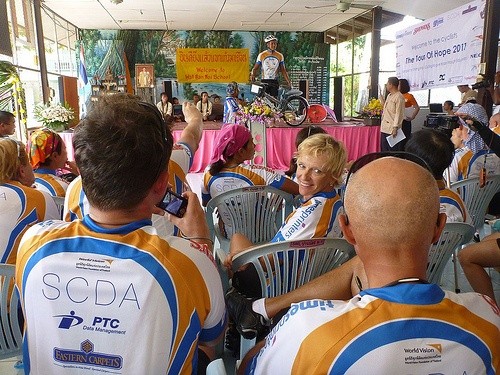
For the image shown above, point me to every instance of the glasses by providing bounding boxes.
[139,101,167,146]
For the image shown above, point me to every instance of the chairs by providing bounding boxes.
[0,171,500,375]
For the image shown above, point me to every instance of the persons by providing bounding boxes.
[192,94,199,106]
[214,96,220,103]
[380,77,420,152]
[225,133,348,300]
[235,156,500,375]
[0,110,80,349]
[196,92,213,121]
[61,100,204,238]
[226,102,500,339]
[172,97,180,105]
[285,124,354,210]
[223,81,241,123]
[457,85,477,108]
[250,34,292,112]
[157,92,173,116]
[201,124,300,239]
[443,100,454,115]
[13,92,227,375]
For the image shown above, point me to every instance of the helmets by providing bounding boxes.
[264,35,278,42]
[226,82,237,95]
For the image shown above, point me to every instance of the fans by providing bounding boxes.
[305,0,373,12]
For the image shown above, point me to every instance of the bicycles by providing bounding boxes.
[248,77,309,127]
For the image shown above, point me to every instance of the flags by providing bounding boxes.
[79,45,88,88]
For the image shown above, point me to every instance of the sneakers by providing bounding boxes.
[225,287,268,340]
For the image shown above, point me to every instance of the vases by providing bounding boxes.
[53,124,65,132]
[364,117,380,126]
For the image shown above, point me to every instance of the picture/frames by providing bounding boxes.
[134,64,154,88]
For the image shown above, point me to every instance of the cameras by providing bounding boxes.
[155,187,188,218]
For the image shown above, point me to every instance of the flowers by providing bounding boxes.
[239,103,283,125]
[360,98,384,117]
[34,102,75,128]
